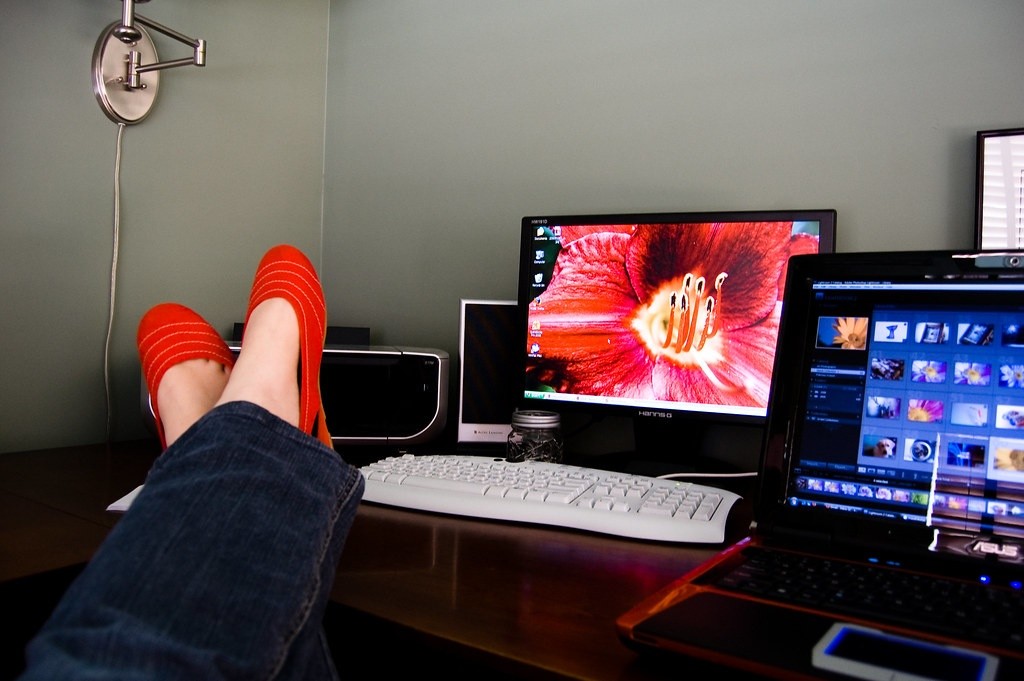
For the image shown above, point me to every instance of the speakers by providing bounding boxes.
[138,343,451,470]
[456,298,517,442]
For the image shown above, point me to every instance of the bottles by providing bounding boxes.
[507,411,562,462]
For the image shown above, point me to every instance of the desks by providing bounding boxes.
[0,440,1024,681]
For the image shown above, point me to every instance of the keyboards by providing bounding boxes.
[352,453,744,548]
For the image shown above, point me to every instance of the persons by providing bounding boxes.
[6,244,365,681]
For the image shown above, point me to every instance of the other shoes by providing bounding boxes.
[136,302,238,451]
[241,245,334,454]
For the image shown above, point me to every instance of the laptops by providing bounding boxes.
[615,250,1024,681]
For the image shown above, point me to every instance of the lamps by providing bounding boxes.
[90,0,207,127]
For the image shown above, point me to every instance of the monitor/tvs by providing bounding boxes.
[515,208,837,489]
